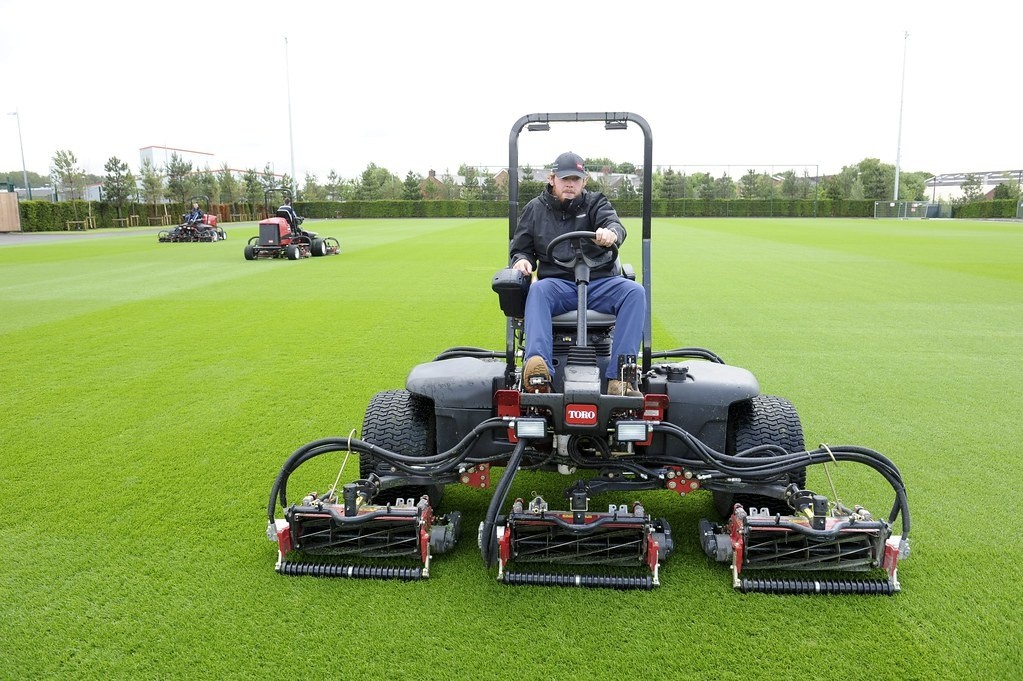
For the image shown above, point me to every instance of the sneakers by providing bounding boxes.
[522,354,552,394]
[606,379,644,397]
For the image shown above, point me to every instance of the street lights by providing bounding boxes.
[8,111,32,200]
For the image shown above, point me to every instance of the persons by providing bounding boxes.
[508,152,649,400]
[185,202,204,225]
[279,197,298,220]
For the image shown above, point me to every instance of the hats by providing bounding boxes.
[553,150,586,179]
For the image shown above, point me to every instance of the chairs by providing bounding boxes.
[190,211,204,224]
[277,209,304,231]
[490,254,638,328]
